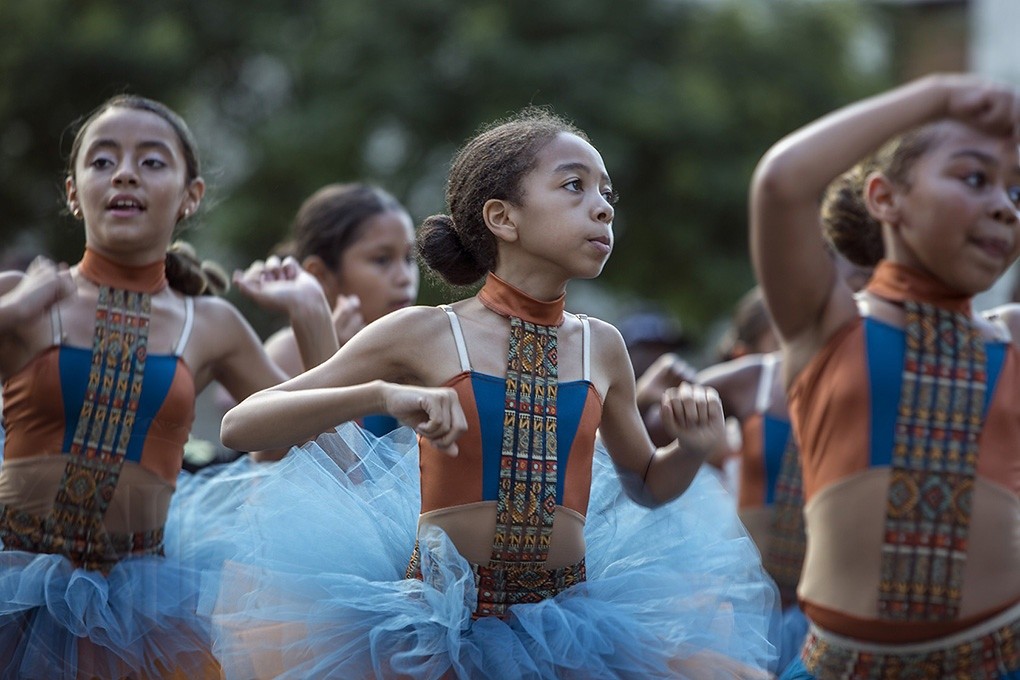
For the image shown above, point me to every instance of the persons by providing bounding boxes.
[217,99,727,678]
[623,255,882,615]
[0,85,342,680]
[217,176,431,484]
[745,65,1020,680]
